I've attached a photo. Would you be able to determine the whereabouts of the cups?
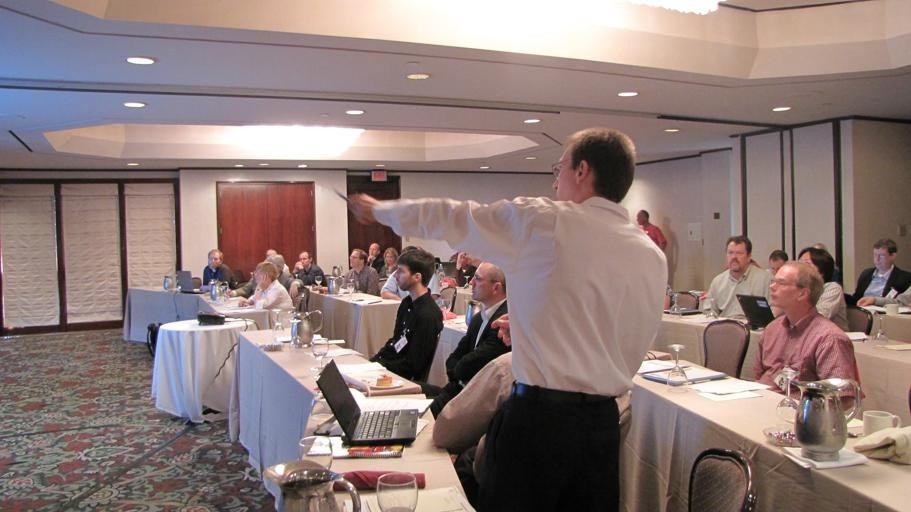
[886,303,902,317]
[863,410,902,438]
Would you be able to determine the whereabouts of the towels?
[782,446,866,468]
[333,473,423,490]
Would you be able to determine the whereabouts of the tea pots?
[465,299,483,327]
[328,276,344,295]
[163,275,174,291]
[291,310,324,349]
[209,279,216,300]
[277,467,364,512]
[332,265,344,278]
[791,377,861,462]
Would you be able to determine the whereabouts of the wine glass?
[216,281,230,305]
[706,297,719,322]
[669,292,682,319]
[668,343,690,395]
[272,308,286,346]
[311,399,333,428]
[873,317,890,348]
[348,283,355,301]
[316,276,322,292]
[376,473,419,512]
[778,367,803,424]
[311,338,328,372]
[463,276,472,291]
[298,435,334,473]
[442,300,452,323]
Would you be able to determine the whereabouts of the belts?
[509,384,610,406]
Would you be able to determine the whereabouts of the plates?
[763,426,802,448]
[369,380,406,388]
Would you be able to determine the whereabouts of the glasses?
[770,280,804,291]
[350,256,364,260]
[552,159,571,179]
[473,274,499,283]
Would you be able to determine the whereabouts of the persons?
[856,284,911,312]
[415,261,518,410]
[202,248,235,291]
[631,207,665,270]
[256,248,287,274]
[289,249,325,288]
[227,256,303,297]
[764,250,792,282]
[851,240,908,306]
[380,244,442,301]
[345,125,664,506]
[365,242,382,273]
[342,248,377,293]
[750,262,862,412]
[702,236,777,326]
[453,247,477,289]
[368,249,442,378]
[797,250,847,331]
[377,247,400,281]
[432,343,632,460]
[239,263,293,334]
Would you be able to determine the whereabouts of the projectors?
[197,311,226,326]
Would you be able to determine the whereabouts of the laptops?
[735,294,775,331]
[316,358,419,446]
[178,272,207,294]
[663,308,703,316]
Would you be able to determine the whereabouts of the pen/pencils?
[332,188,348,201]
[682,378,710,385]
[368,300,382,304]
[352,299,364,301]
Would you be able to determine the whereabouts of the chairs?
[670,291,699,310]
[847,304,873,335]
[192,277,202,288]
[703,319,750,379]
[440,287,457,312]
[377,278,387,290]
[298,286,310,309]
[292,292,307,312]
[688,447,758,511]
[236,281,249,288]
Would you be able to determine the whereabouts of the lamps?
[626,0,728,16]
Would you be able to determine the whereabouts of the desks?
[229,329,422,481]
[698,298,711,311]
[302,394,476,512]
[302,285,402,360]
[620,359,911,511]
[151,318,257,424]
[873,313,911,344]
[123,285,206,344]
[651,309,911,428]
[427,315,469,389]
[439,286,473,315]
[198,294,270,330]
